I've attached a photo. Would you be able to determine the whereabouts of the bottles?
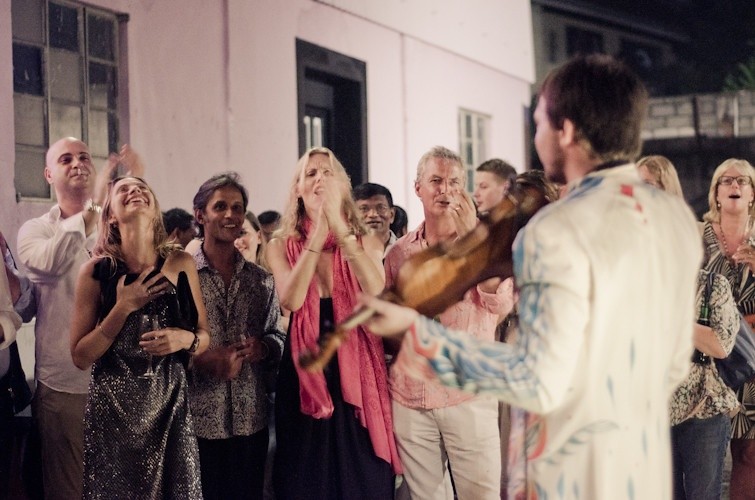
[693,303,711,364]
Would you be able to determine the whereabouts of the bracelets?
[99,325,118,340]
[301,247,324,255]
[335,229,356,242]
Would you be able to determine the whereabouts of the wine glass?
[138,315,162,380]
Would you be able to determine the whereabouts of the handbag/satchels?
[176,271,199,368]
[706,271,755,388]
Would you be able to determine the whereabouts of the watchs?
[185,331,199,352]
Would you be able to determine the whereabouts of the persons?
[357,51,704,500]
[0,134,755,500]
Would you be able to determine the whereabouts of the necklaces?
[717,216,748,265]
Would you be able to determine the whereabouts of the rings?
[147,290,152,295]
[454,207,461,211]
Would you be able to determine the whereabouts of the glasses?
[717,175,754,188]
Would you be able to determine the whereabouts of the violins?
[300,194,548,373]
